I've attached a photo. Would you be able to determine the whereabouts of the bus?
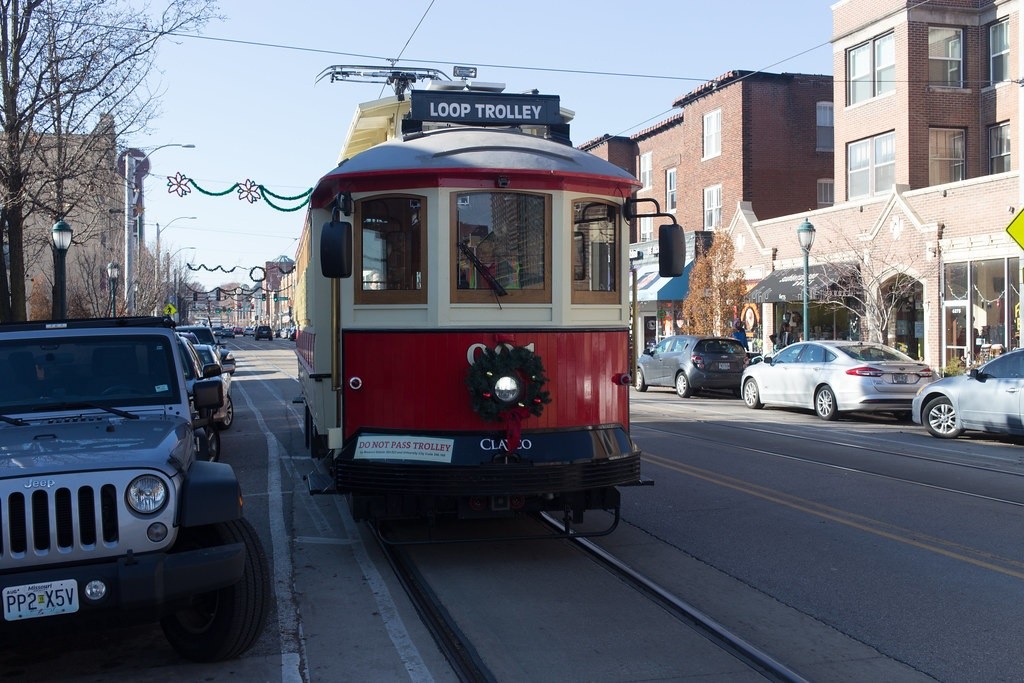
[290,59,685,544]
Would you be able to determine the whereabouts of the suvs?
[0,315,271,663]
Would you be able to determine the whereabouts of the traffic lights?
[215,308,220,315]
[237,303,241,309]
[274,293,278,301]
[251,304,254,309]
[227,307,230,313]
[262,293,266,301]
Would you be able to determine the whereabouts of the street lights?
[173,264,197,324]
[125,143,197,317]
[50,218,72,323]
[796,218,817,341]
[155,216,198,318]
[107,259,119,319]
[166,247,196,314]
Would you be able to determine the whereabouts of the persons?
[732,318,749,352]
[461,242,516,290]
[775,322,793,349]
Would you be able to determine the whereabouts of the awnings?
[628,257,694,302]
[743,262,862,303]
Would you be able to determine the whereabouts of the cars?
[274,326,296,341]
[912,348,1024,439]
[152,322,274,463]
[635,335,752,400]
[741,340,933,421]
